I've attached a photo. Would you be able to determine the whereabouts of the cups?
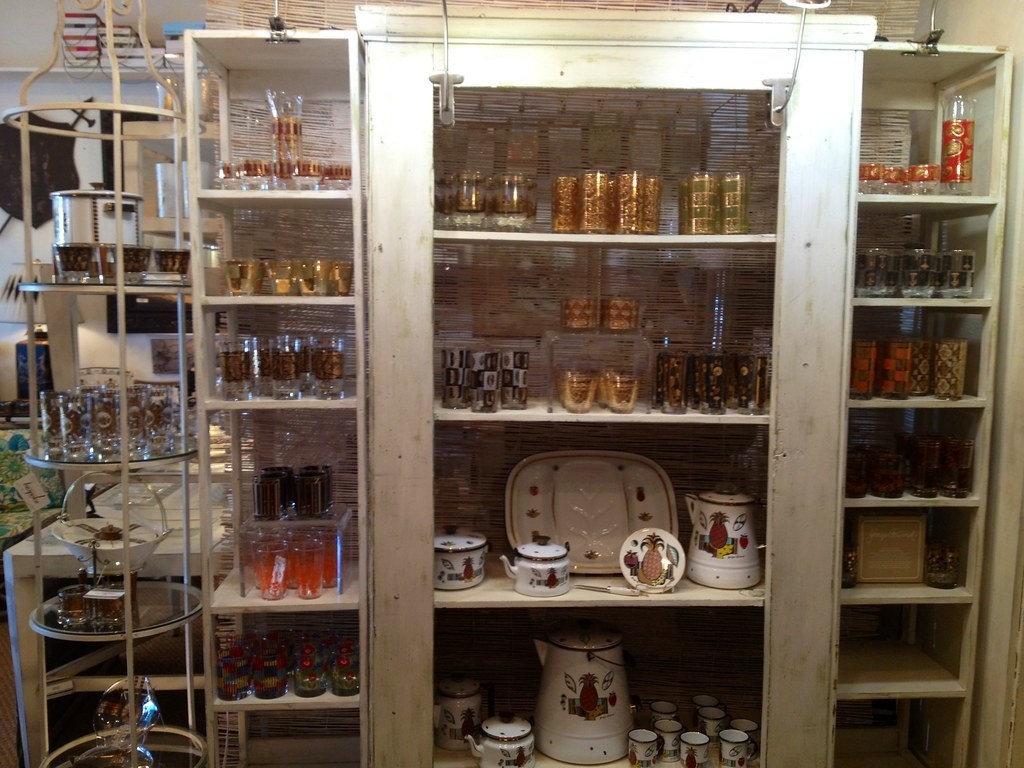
[842,548,858,587]
[214,330,346,400]
[216,626,360,701]
[219,88,351,190]
[852,243,980,297]
[248,465,333,600]
[625,694,762,768]
[56,569,139,631]
[40,379,177,458]
[218,250,355,297]
[923,539,962,590]
[434,164,750,235]
[856,93,979,195]
[52,240,190,286]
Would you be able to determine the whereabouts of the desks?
[3,443,257,768]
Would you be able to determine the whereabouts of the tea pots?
[464,710,536,768]
[683,479,767,590]
[498,536,571,597]
[433,672,493,751]
[529,617,640,765]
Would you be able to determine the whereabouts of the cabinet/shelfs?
[366,34,1013,768]
[0,0,366,768]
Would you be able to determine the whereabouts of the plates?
[620,528,686,594]
[505,450,679,576]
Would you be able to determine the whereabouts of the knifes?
[575,584,641,597]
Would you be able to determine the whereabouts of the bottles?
[848,337,971,400]
[548,294,653,412]
[438,343,769,415]
[846,426,978,501]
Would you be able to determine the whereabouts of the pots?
[434,523,492,590]
[47,181,145,249]
